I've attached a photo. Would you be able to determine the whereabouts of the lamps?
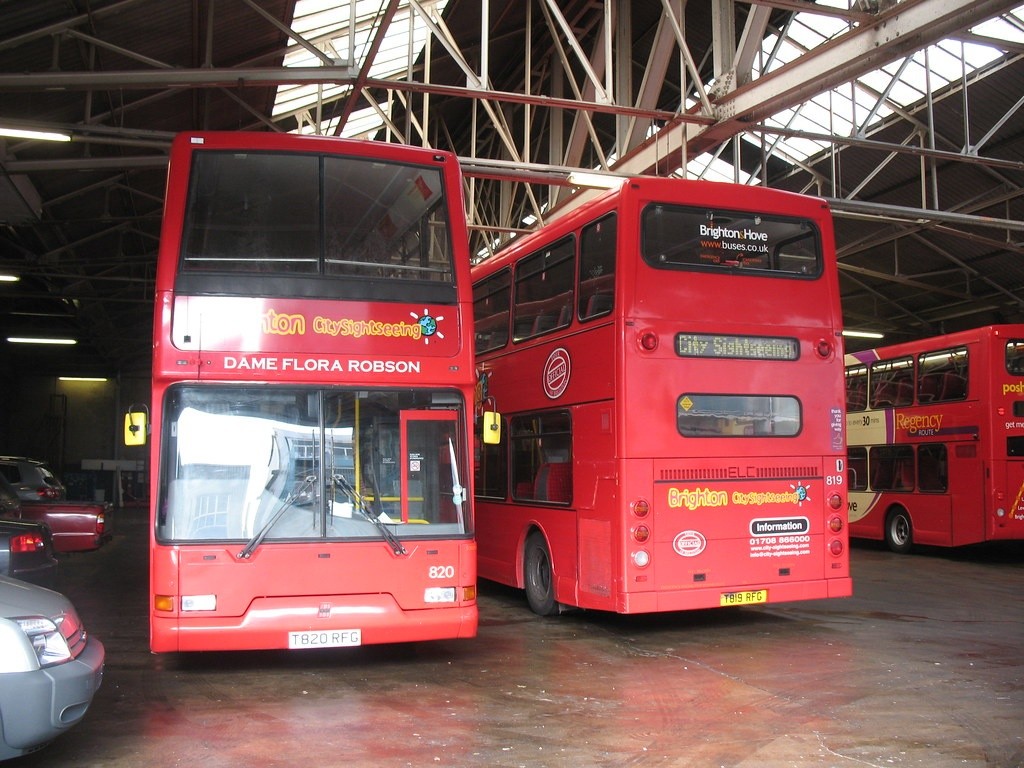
[567,172,625,191]
[0,122,73,142]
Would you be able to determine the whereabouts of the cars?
[0,454,116,762]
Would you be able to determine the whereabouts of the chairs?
[847,455,945,489]
[516,459,573,502]
[475,274,615,352]
[847,371,966,410]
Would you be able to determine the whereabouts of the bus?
[468,177,854,618]
[844,323,1024,556]
[122,130,483,652]
